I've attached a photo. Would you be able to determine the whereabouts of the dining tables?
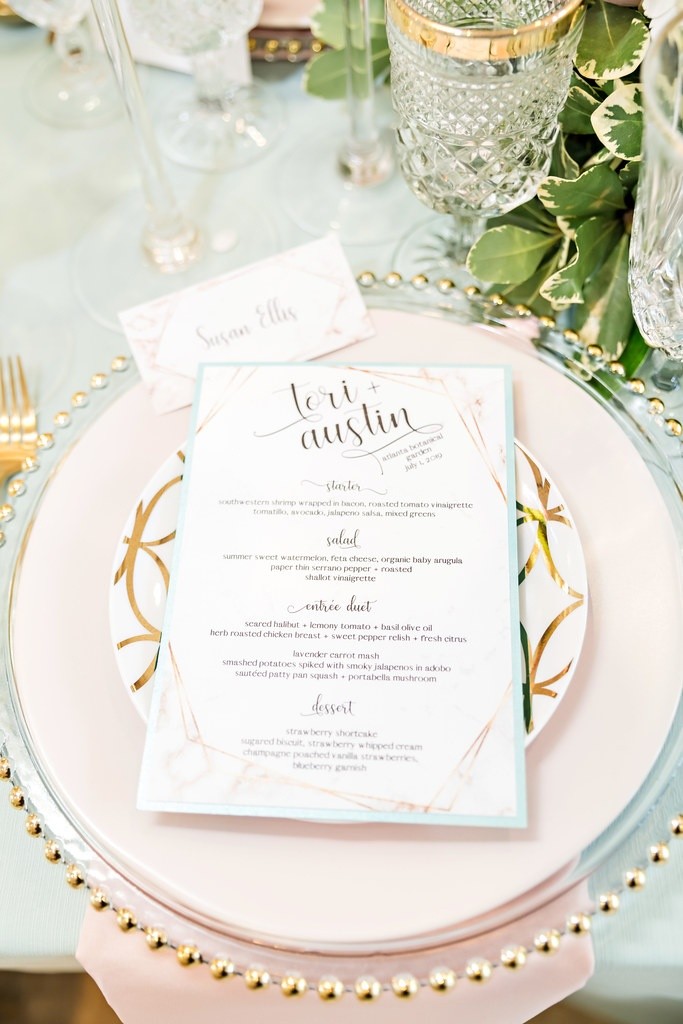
[0,1,683,1024]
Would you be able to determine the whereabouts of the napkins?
[4,1,263,86]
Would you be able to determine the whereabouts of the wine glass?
[4,1,131,128]
[628,0,683,406]
[385,0,589,292]
[123,0,286,173]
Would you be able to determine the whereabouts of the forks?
[0,354,38,495]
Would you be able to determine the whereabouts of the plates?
[7,309,682,960]
[108,436,592,754]
[2,269,682,1003]
